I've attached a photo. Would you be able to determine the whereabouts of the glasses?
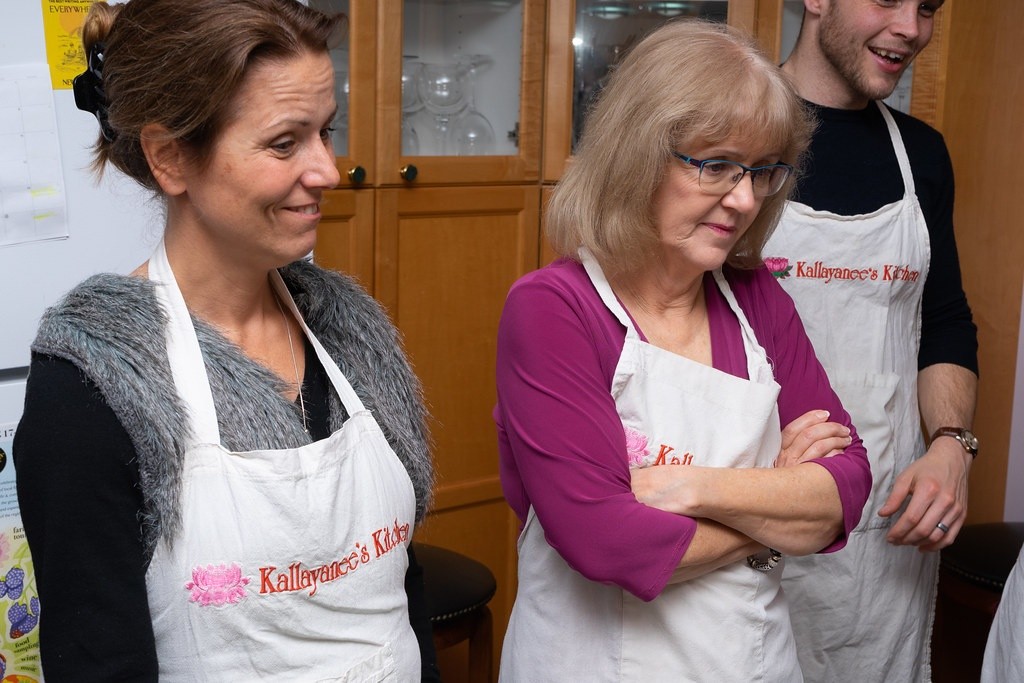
[669,148,795,198]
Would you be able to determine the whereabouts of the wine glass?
[329,52,497,156]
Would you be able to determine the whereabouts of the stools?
[399,543,499,683]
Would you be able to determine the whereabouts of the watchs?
[927,425,978,461]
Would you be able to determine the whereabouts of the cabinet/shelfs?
[294,0,732,683]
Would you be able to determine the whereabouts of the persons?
[11,0,441,683]
[493,13,871,683]
[758,1,977,683]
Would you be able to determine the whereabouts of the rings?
[936,522,949,532]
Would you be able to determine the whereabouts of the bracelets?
[746,548,781,573]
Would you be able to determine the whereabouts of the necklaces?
[269,289,311,435]
